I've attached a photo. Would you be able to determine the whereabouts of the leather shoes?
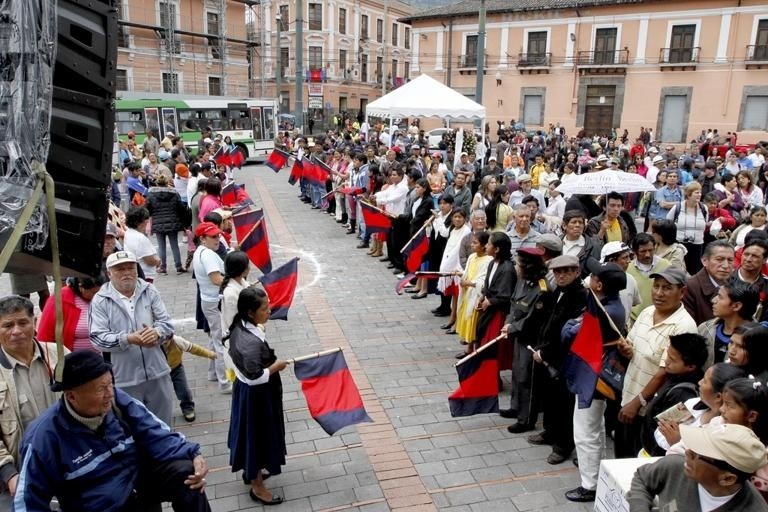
[431,306,469,359]
[249,487,283,505]
[242,468,271,484]
[565,486,596,501]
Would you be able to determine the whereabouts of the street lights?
[276,10,281,97]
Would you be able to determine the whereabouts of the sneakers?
[184,410,195,422]
[298,194,428,299]
[547,446,572,464]
[527,431,551,444]
[208,375,218,381]
[499,408,520,420]
[220,384,233,394]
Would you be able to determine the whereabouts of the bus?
[112,96,281,165]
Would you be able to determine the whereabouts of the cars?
[277,113,295,130]
[424,128,455,149]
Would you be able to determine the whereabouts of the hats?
[106,250,139,269]
[611,158,620,165]
[548,255,580,270]
[597,154,609,162]
[430,152,441,158]
[159,150,172,161]
[679,423,768,473]
[204,125,220,145]
[516,174,532,186]
[652,155,666,165]
[533,233,563,252]
[488,157,496,162]
[51,349,112,392]
[587,257,627,290]
[647,146,658,154]
[128,130,175,138]
[599,241,629,263]
[105,224,117,237]
[516,247,544,259]
[648,265,689,286]
[460,152,468,158]
[194,222,222,237]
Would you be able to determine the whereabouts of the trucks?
[695,134,756,157]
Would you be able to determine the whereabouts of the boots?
[156,264,168,276]
[175,264,189,275]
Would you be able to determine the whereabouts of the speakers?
[0,0,119,94]
[0,181,110,280]
[0,81,114,185]
